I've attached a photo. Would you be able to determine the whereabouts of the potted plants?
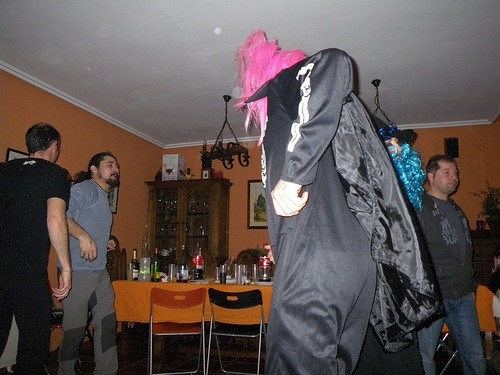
[469,180,500,229]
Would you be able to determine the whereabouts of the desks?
[111,277,274,375]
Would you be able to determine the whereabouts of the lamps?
[200,94,252,170]
[367,79,419,145]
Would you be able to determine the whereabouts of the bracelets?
[62,268,73,273]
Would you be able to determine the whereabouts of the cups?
[233,263,248,285]
[250,263,259,282]
[127,263,134,281]
[169,264,177,282]
[219,264,227,284]
[157,198,208,235]
[176,263,189,282]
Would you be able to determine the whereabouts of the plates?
[190,277,275,284]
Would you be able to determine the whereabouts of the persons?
[56,152,119,375]
[418,154,485,375]
[0,122,71,375]
[105,235,120,282]
[372,115,442,375]
[231,30,439,375]
[442,249,500,375]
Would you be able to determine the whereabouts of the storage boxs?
[200,170,224,179]
[161,154,183,180]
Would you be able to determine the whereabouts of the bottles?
[259,243,272,282]
[151,248,161,283]
[139,243,153,282]
[130,248,140,281]
[193,247,203,281]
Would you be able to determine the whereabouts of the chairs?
[145,286,206,375]
[206,287,267,375]
[43,304,95,375]
[436,284,496,375]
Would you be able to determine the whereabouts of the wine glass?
[213,266,220,282]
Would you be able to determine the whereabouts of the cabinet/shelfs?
[470,229,500,294]
[143,179,235,275]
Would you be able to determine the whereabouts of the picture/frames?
[6,148,29,161]
[247,179,271,229]
[108,185,120,214]
